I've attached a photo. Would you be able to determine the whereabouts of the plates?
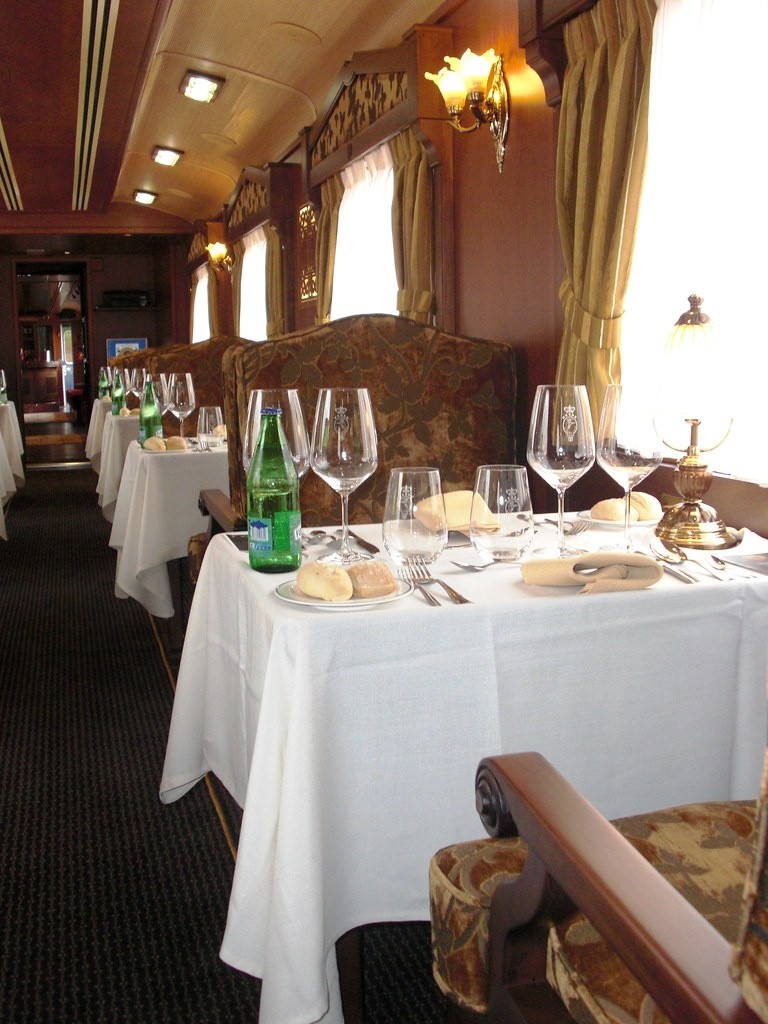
[274,578,413,612]
[146,450,186,452]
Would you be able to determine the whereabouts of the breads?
[143,436,188,451]
[213,424,227,439]
[119,408,140,417]
[296,560,397,600]
[102,396,110,400]
[590,492,663,522]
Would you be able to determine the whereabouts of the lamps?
[132,189,160,207]
[650,294,741,550]
[204,242,231,272]
[150,144,184,168]
[423,48,509,175]
[177,69,226,104]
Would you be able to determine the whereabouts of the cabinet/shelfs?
[19,315,65,412]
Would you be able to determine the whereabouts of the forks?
[543,516,594,536]
[397,568,441,606]
[201,442,208,451]
[407,556,469,604]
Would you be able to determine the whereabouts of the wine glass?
[241,389,310,564]
[166,373,196,449]
[526,384,595,559]
[309,388,378,566]
[595,384,663,554]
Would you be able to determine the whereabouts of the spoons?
[451,559,498,573]
[312,530,337,542]
[650,538,725,582]
[707,553,751,579]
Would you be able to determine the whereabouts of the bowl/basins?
[575,509,665,532]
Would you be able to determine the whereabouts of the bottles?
[246,408,302,573]
[98,370,109,399]
[139,382,163,448]
[111,374,126,415]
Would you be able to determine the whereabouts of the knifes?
[337,529,379,554]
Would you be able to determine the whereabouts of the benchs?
[434,736,768,1024]
[108,311,530,588]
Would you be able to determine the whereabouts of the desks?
[93,409,141,525]
[103,433,229,619]
[154,506,768,1024]
[0,401,26,540]
[84,396,112,475]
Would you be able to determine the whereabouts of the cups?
[197,406,223,446]
[470,464,534,563]
[383,466,448,566]
[100,366,170,416]
